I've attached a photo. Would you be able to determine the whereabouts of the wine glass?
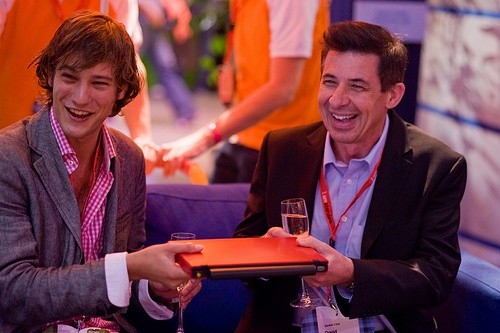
[171,232,196,333]
[281,198,323,307]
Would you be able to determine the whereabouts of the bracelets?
[207,121,222,143]
[342,274,354,293]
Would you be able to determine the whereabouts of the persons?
[0,0,201,184]
[233,20,468,333]
[0,8,202,333]
[158,0,331,184]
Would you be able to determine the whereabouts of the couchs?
[125,183,500,333]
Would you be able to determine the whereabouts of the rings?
[176,283,184,292]
